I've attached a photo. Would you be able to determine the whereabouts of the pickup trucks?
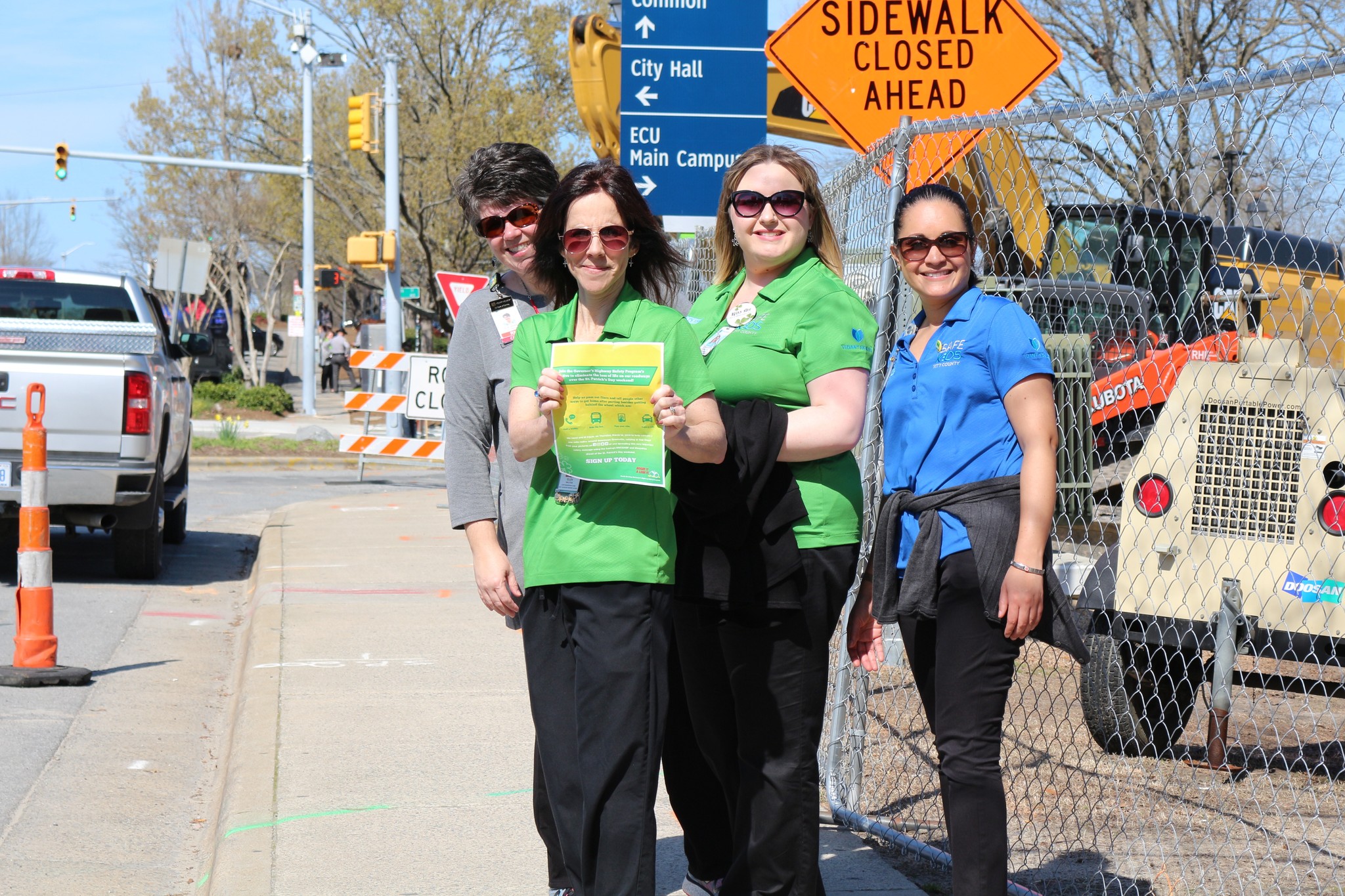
[0,265,212,579]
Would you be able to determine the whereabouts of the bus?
[148,258,243,363]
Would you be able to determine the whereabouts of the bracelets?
[1010,560,1045,575]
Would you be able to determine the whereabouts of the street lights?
[1212,150,1246,228]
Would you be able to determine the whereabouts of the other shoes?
[548,887,574,896]
[667,871,722,896]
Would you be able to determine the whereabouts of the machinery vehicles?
[572,15,1345,506]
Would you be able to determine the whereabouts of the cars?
[244,318,283,356]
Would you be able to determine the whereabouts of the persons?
[319,303,333,332]
[508,159,727,896]
[846,184,1059,896]
[329,328,361,392]
[662,144,877,896]
[445,142,690,896]
[320,330,336,393]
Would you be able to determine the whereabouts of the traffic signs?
[619,0,770,235]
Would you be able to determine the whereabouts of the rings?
[670,406,675,415]
[534,390,544,400]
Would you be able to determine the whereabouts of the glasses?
[476,203,546,239]
[560,224,639,255]
[897,231,974,262]
[725,190,813,218]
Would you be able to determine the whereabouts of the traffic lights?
[347,93,371,153]
[320,269,340,288]
[54,143,69,180]
[381,234,395,261]
[71,206,78,220]
